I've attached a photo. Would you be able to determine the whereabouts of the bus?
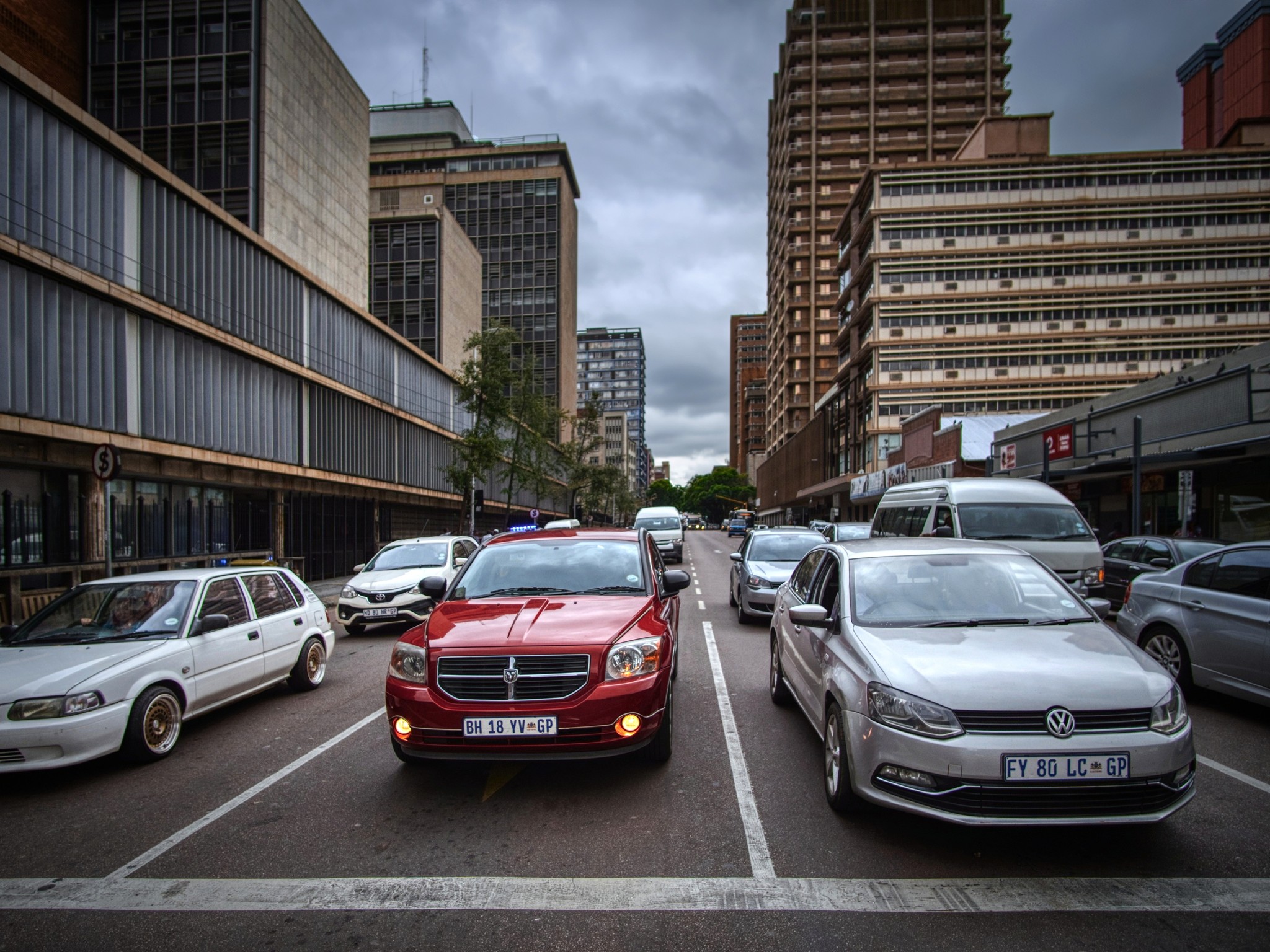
[733,510,756,532]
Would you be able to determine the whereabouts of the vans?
[870,477,1111,619]
[544,519,582,529]
[633,506,687,563]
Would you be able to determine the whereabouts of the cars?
[383,527,691,768]
[1100,535,1226,609]
[754,520,873,542]
[768,537,1199,826]
[0,566,334,776]
[1113,541,1270,707]
[688,521,720,530]
[336,535,480,635]
[721,519,729,531]
[729,528,830,624]
[1218,494,1270,540]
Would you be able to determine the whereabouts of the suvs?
[728,519,748,537]
